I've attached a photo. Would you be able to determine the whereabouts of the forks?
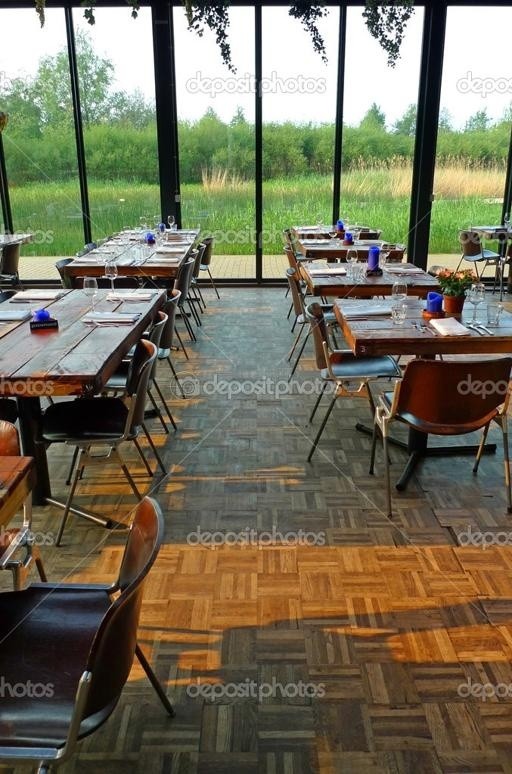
[420,320,438,336]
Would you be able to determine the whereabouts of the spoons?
[410,319,427,333]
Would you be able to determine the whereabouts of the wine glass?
[328,225,339,248]
[153,215,161,230]
[139,216,148,231]
[345,248,358,278]
[391,281,408,307]
[316,213,325,231]
[83,277,99,319]
[97,225,131,264]
[466,282,486,325]
[104,262,119,295]
[504,212,512,225]
[158,222,166,242]
[167,215,175,231]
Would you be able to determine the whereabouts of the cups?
[389,304,408,325]
[134,227,141,243]
[351,265,364,281]
[135,243,151,261]
[486,304,503,325]
